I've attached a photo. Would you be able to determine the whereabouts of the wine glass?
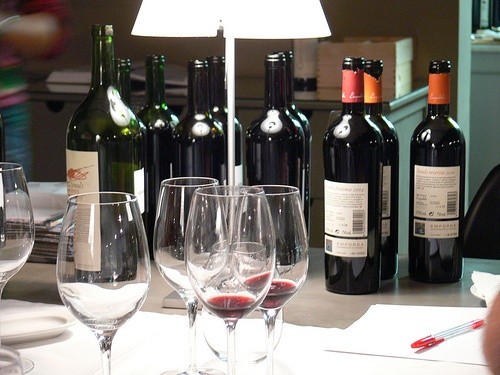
[183,186,276,375]
[232,184,310,374]
[152,177,224,375]
[0,162,35,375]
[56,192,152,375]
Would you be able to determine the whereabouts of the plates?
[0,311,71,345]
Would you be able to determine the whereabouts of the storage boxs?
[316,36,414,101]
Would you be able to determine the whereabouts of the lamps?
[130,0,332,239]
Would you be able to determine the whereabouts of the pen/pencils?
[411,318,485,349]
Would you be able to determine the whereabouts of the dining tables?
[0,246,500,375]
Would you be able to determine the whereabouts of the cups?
[201,241,284,367]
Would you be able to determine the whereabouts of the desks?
[28,64,429,246]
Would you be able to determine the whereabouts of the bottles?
[321,56,383,296]
[114,53,311,277]
[64,24,138,284]
[362,58,400,281]
[409,59,466,285]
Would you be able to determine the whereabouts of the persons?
[0,0,69,185]
